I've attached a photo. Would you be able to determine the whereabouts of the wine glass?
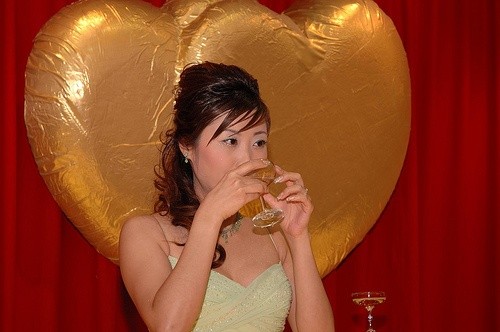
[352,292,387,332]
[242,159,285,229]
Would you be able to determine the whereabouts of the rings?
[302,186,309,196]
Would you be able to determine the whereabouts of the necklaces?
[217,212,244,240]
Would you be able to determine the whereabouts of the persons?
[116,58,339,331]
